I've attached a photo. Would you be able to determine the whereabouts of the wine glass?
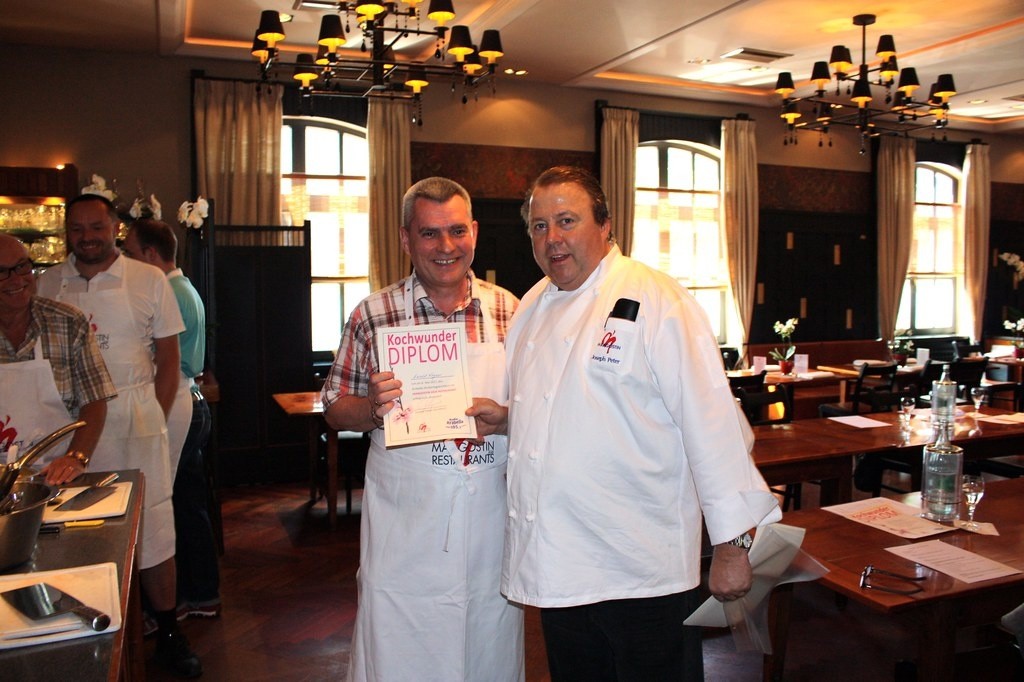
[901,397,915,422]
[960,474,985,530]
[970,387,985,418]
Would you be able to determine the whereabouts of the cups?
[753,356,767,372]
[916,348,930,367]
[0,204,67,279]
[794,354,809,375]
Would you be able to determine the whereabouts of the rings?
[374,399,382,406]
[69,466,74,470]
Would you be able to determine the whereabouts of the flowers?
[878,336,914,350]
[1003,319,1024,346]
[767,316,800,360]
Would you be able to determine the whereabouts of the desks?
[267,353,1022,681]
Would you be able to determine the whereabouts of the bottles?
[920,365,964,522]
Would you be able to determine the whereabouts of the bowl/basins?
[0,464,60,570]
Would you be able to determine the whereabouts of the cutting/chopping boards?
[41,481,134,525]
[0,561,122,648]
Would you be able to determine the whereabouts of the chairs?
[903,355,962,405]
[817,362,898,416]
[949,356,989,403]
[730,383,789,425]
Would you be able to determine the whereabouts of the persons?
[125,219,219,633]
[466,166,782,682]
[0,233,119,484]
[319,177,522,682]
[37,194,201,682]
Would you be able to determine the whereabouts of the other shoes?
[143,601,187,635]
[187,594,220,617]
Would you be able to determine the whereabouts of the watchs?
[725,533,754,549]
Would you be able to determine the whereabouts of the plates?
[853,359,887,366]
[751,365,782,371]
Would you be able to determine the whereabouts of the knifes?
[42,519,105,527]
[1,582,111,631]
[53,473,119,511]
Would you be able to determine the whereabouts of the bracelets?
[65,450,90,468]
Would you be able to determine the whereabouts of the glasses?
[859,564,928,594]
[0,257,34,282]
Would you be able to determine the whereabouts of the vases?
[893,352,909,364]
[1013,347,1024,358]
[777,359,793,375]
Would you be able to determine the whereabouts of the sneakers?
[154,633,205,677]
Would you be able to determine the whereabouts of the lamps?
[249,0,504,127]
[774,13,958,157]
